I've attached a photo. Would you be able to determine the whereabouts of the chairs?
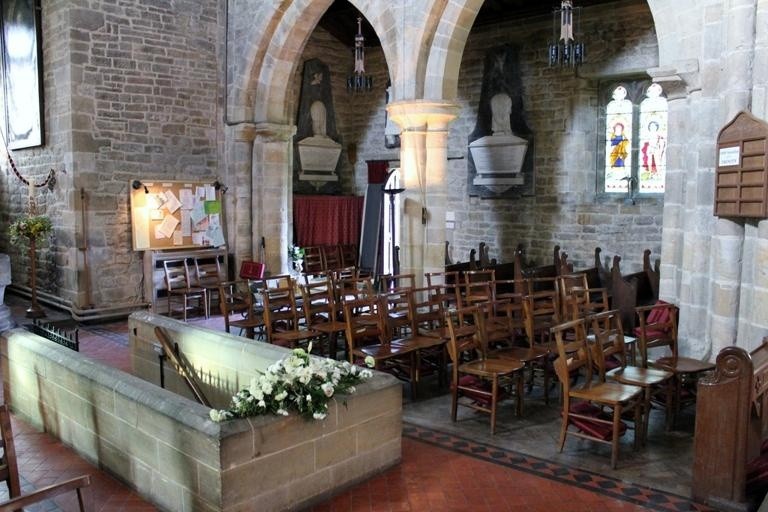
[0,406,25,512]
[0,475,92,512]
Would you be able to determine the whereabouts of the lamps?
[549,0,588,67]
[346,17,373,93]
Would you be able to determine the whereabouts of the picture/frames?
[0,0,45,151]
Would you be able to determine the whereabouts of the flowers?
[6,215,53,246]
